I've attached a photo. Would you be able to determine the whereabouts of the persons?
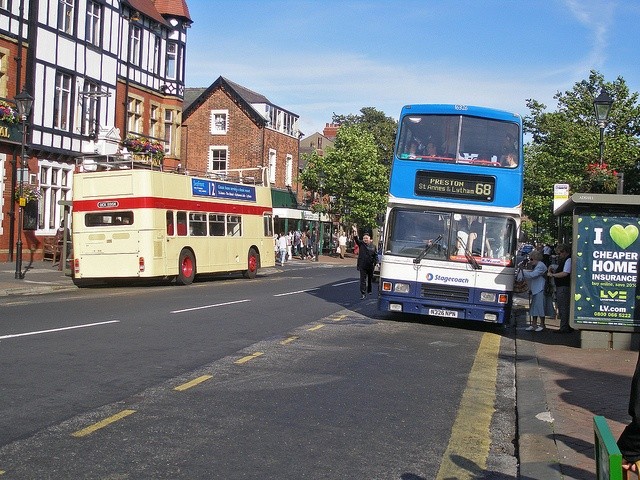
[518,251,549,333]
[547,243,575,335]
[339,234,347,259]
[278,232,288,267]
[288,227,338,268]
[454,220,468,256]
[351,226,379,301]
[467,221,493,259]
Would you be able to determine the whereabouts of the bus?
[378,104,523,323]
[72,152,275,287]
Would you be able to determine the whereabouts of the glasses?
[530,258,534,261]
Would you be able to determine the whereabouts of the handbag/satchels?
[513,264,528,294]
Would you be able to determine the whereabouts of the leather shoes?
[568,328,574,332]
[525,325,538,331]
[535,325,546,331]
[553,328,567,334]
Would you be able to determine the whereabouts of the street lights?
[594,88,614,170]
[13,85,34,279]
